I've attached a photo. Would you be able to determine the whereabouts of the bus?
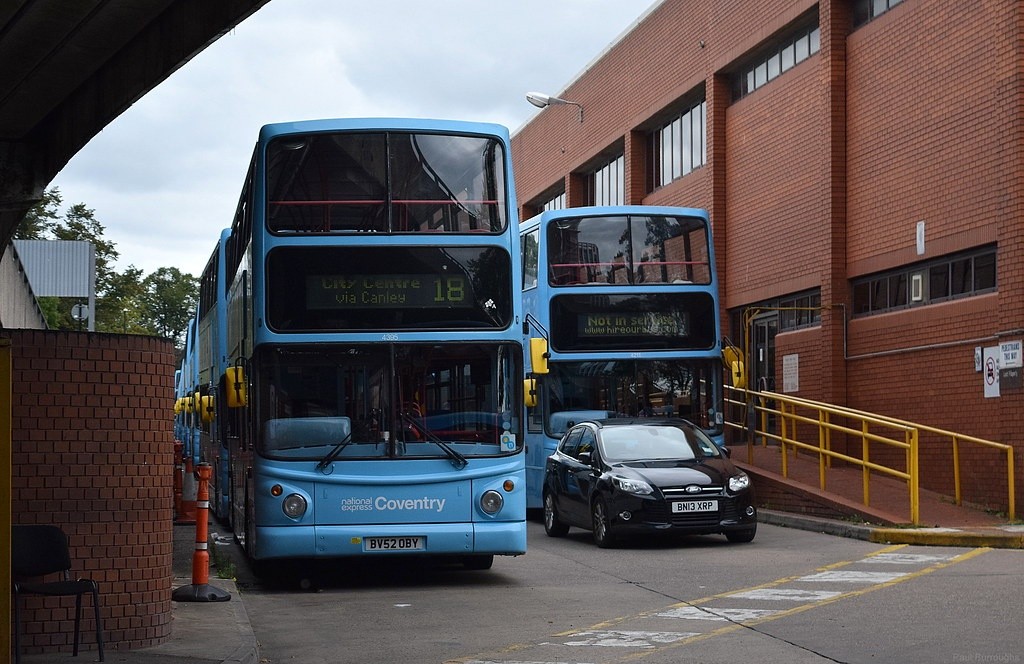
[430,207,745,510]
[175,118,549,570]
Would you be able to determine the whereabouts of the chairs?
[10,523,105,664]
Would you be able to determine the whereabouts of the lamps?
[526,92,583,123]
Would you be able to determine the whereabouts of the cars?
[542,418,758,547]
